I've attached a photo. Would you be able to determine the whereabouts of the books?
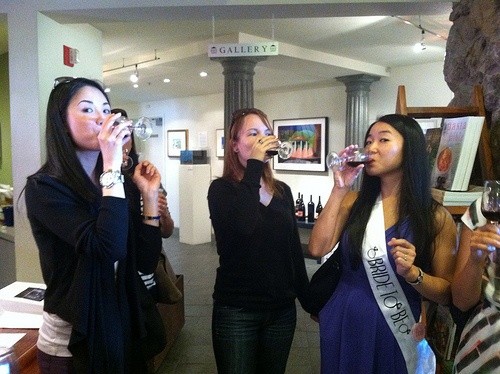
[430,115,485,191]
[431,184,492,207]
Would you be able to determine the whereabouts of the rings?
[403,253,408,260]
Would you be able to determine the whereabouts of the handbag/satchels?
[308,250,344,317]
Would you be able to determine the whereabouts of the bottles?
[316,195,322,220]
[296,193,300,218]
[298,194,305,221]
[308,195,314,222]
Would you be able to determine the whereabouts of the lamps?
[103,83,110,93]
[130,64,139,83]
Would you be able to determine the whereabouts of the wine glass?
[326,147,370,170]
[112,116,152,142]
[120,148,133,171]
[266,138,293,159]
[481,180,500,252]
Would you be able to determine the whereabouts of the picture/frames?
[216,128,225,157]
[166,130,188,157]
[272,116,328,172]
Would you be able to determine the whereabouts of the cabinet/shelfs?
[294,216,325,264]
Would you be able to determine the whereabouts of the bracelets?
[406,267,425,287]
[141,214,161,221]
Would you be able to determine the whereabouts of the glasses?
[229,108,267,139]
[53,76,104,90]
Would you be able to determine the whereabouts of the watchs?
[99,168,125,189]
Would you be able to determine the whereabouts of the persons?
[450,189,500,374]
[110,108,174,239]
[15,74,162,374]
[205,106,325,374]
[308,111,457,374]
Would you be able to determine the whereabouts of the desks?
[0,327,42,374]
